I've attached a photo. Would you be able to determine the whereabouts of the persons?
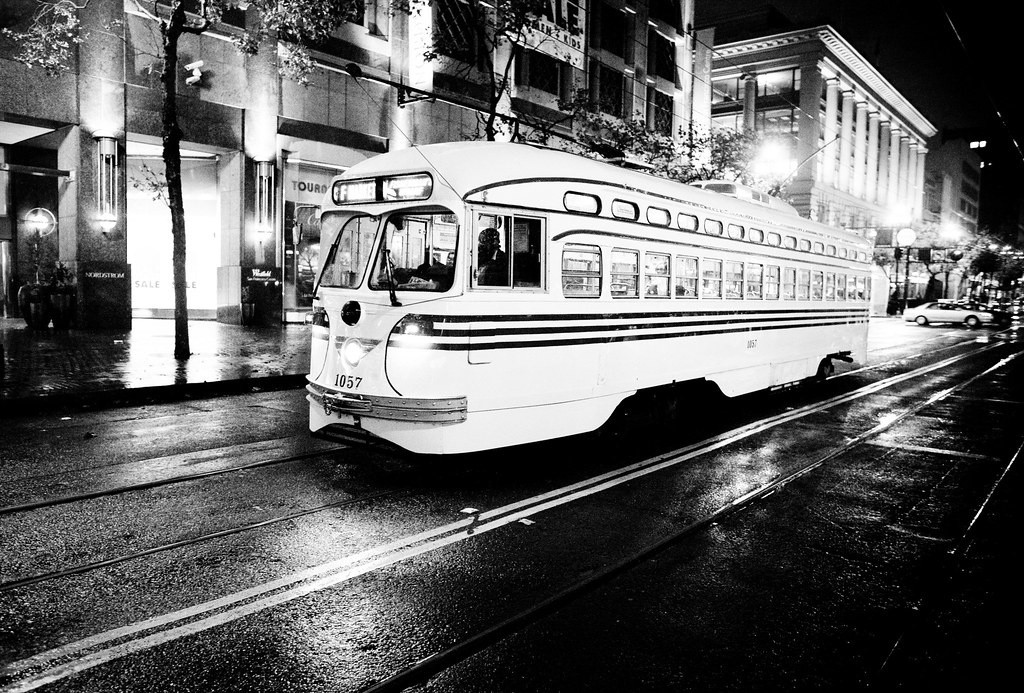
[446,252,455,267]
[477,228,508,287]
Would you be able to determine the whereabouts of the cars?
[962,295,1024,328]
[902,301,994,329]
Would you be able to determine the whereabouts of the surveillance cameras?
[184,60,204,71]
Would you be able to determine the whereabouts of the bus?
[305,143,874,468]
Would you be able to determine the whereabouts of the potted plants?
[18,229,74,334]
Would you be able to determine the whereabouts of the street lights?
[896,227,917,314]
